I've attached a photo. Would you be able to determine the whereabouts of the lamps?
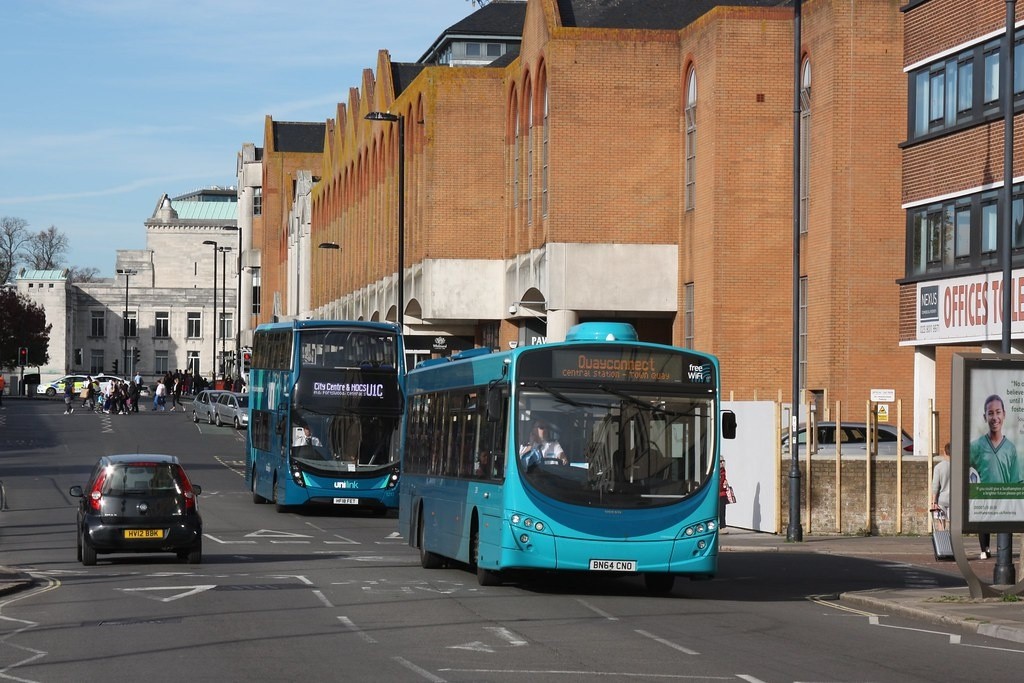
[318,243,342,250]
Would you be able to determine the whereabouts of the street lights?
[212,247,232,380]
[364,111,404,329]
[116,269,137,373]
[202,240,217,390]
[223,225,242,392]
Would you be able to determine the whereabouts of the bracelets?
[934,502,937,504]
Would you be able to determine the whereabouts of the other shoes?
[71,409,75,414]
[64,411,69,415]
[985,546,990,553]
[719,528,729,534]
[152,408,157,411]
[81,402,140,415]
[169,408,176,412]
[981,552,987,560]
[182,404,186,412]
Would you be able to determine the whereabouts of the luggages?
[929,508,955,562]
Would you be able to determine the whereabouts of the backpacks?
[520,448,544,473]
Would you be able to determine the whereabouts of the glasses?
[537,424,551,430]
[719,460,725,463]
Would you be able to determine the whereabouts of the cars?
[191,381,249,430]
[140,385,152,397]
[69,454,202,566]
[782,422,913,455]
[37,373,130,397]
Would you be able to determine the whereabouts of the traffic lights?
[18,347,28,366]
[230,350,236,368]
[112,359,118,374]
[75,349,81,365]
[244,353,251,373]
[135,347,141,363]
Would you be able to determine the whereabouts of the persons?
[163,368,209,395]
[134,372,142,392]
[169,377,186,411]
[477,449,490,474]
[970,394,1019,484]
[234,375,246,392]
[63,381,74,414]
[0,373,6,406]
[295,425,322,446]
[719,455,729,534]
[223,375,234,390]
[519,420,569,466]
[932,443,951,531]
[151,380,166,411]
[150,468,173,487]
[81,376,140,414]
[979,533,990,560]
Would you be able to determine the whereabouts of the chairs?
[632,441,664,480]
[370,360,379,367]
[359,360,373,369]
[379,360,393,368]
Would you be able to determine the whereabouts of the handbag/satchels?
[157,394,166,405]
[79,388,89,398]
[723,480,736,503]
[124,389,129,400]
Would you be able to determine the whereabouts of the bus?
[398,322,738,587]
[245,320,406,514]
